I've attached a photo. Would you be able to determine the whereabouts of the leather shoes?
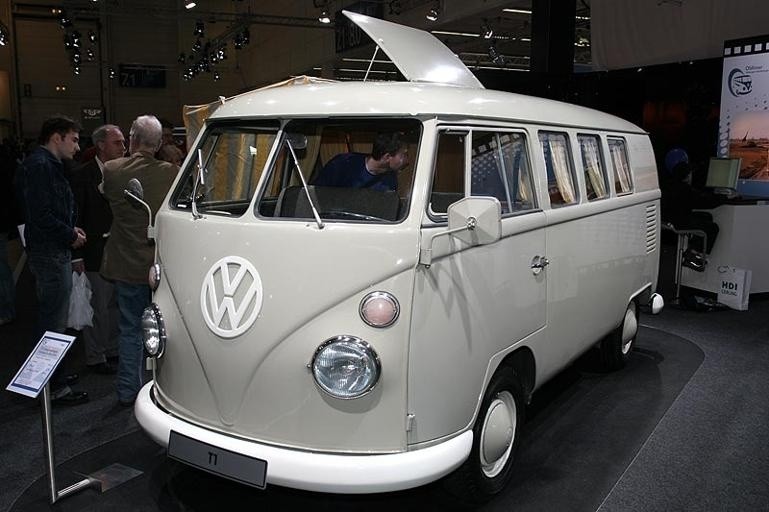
[49,370,90,407]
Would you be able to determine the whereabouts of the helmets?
[662,148,688,178]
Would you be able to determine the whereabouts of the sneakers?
[681,249,709,273]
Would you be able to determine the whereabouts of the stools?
[660,221,710,307]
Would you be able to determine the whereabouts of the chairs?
[270,182,486,224]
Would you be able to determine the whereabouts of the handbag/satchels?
[66,269,97,333]
[716,264,753,313]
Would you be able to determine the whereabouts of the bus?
[132,10,666,498]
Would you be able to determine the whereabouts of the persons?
[313,131,409,192]
[104,115,182,406]
[1,143,16,326]
[70,125,127,374]
[160,144,184,167]
[154,120,175,160]
[18,115,89,406]
[659,163,737,271]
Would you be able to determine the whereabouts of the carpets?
[7,321,745,512]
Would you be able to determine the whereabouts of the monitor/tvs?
[704,157,741,194]
